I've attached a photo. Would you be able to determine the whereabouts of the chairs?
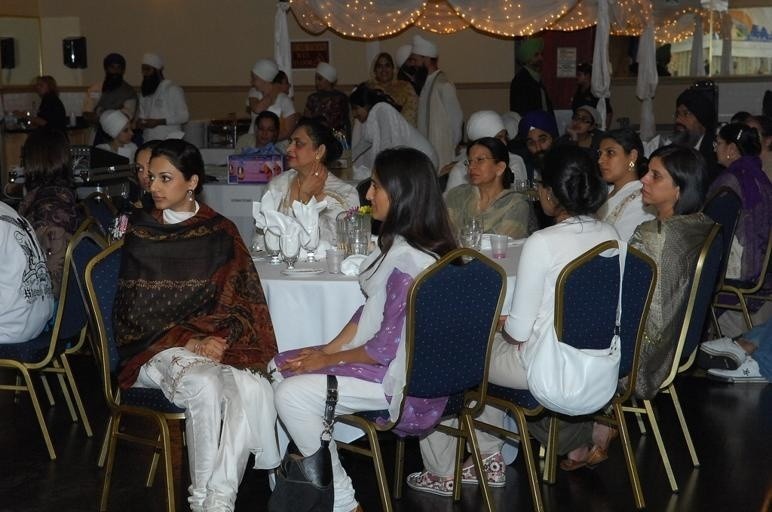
[0,148,771,512]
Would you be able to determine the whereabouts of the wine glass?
[251,226,322,276]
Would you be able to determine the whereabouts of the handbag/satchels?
[267,373,339,512]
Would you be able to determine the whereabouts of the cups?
[488,234,508,259]
[327,248,345,273]
[459,215,482,255]
[516,177,540,198]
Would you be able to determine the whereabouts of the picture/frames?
[289,38,331,71]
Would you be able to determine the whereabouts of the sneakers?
[406,469,455,497]
[557,414,620,472]
[461,451,507,488]
[700,336,748,368]
[706,355,769,384]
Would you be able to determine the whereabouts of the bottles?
[4,102,40,127]
[69,112,77,126]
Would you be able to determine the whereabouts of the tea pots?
[335,209,371,256]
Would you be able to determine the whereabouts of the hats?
[251,58,279,83]
[466,110,506,142]
[502,110,521,141]
[315,61,338,84]
[515,38,544,64]
[574,105,602,129]
[517,113,555,147]
[103,53,127,75]
[411,34,440,58]
[394,44,413,70]
[141,52,165,71]
[99,109,129,138]
[675,88,713,130]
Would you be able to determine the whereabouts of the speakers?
[0,37,15,69]
[62,36,87,68]
